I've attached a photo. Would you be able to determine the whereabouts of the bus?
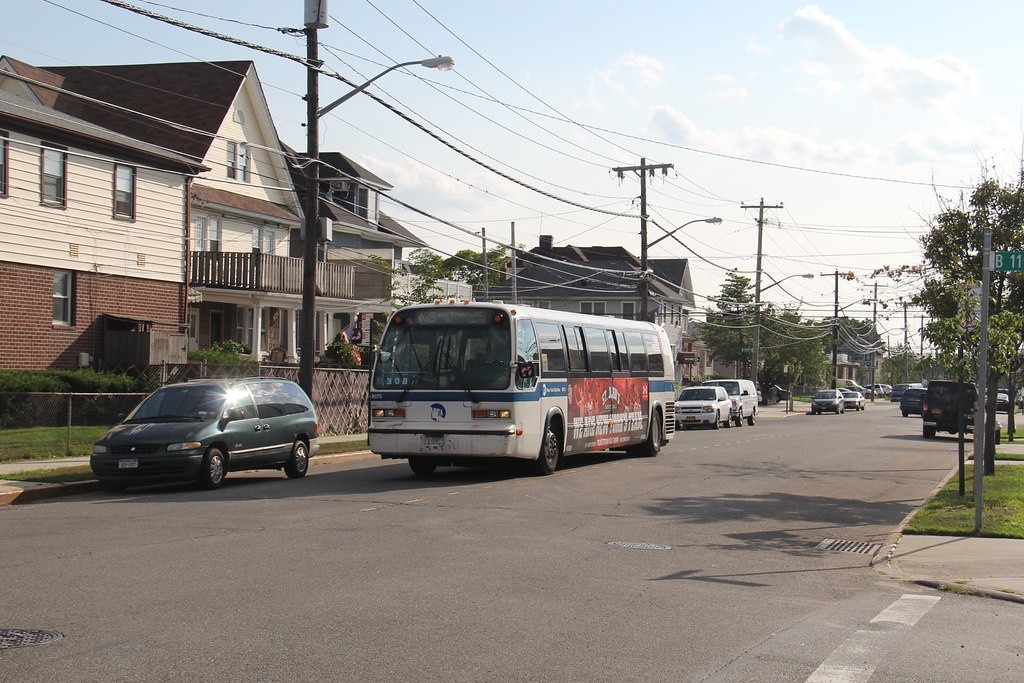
[354,300,676,475]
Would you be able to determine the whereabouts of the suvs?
[675,385,733,429]
[923,379,979,437]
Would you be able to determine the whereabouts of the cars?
[846,387,877,399]
[900,388,928,417]
[842,392,866,411]
[998,389,1009,394]
[866,384,892,395]
[756,382,790,405]
[809,389,845,415]
[984,393,1010,413]
[837,388,852,393]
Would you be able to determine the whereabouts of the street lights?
[638,217,722,319]
[752,272,814,384]
[870,328,904,402]
[297,54,455,397]
[832,298,867,388]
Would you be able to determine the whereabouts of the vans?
[701,380,759,427]
[90,376,320,488]
[891,383,923,402]
